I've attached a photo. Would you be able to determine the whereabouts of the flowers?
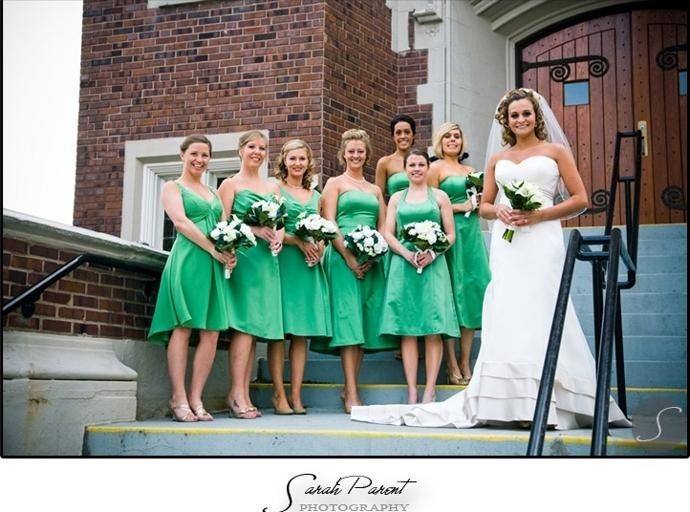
[400,218,450,254]
[344,224,388,261]
[497,179,541,242]
[296,210,338,242]
[247,199,286,231]
[211,220,262,254]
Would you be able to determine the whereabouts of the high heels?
[272,397,295,414]
[168,397,199,422]
[193,403,213,421]
[339,390,366,414]
[287,394,306,414]
[225,395,262,419]
[445,364,470,385]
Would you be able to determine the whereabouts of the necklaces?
[286,180,304,189]
[344,171,366,184]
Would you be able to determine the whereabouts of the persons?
[319,127,388,415]
[160,133,238,422]
[218,130,286,422]
[464,87,633,432]
[428,121,491,387]
[384,148,457,405]
[374,114,430,361]
[268,137,326,417]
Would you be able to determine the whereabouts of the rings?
[525,219,528,224]
